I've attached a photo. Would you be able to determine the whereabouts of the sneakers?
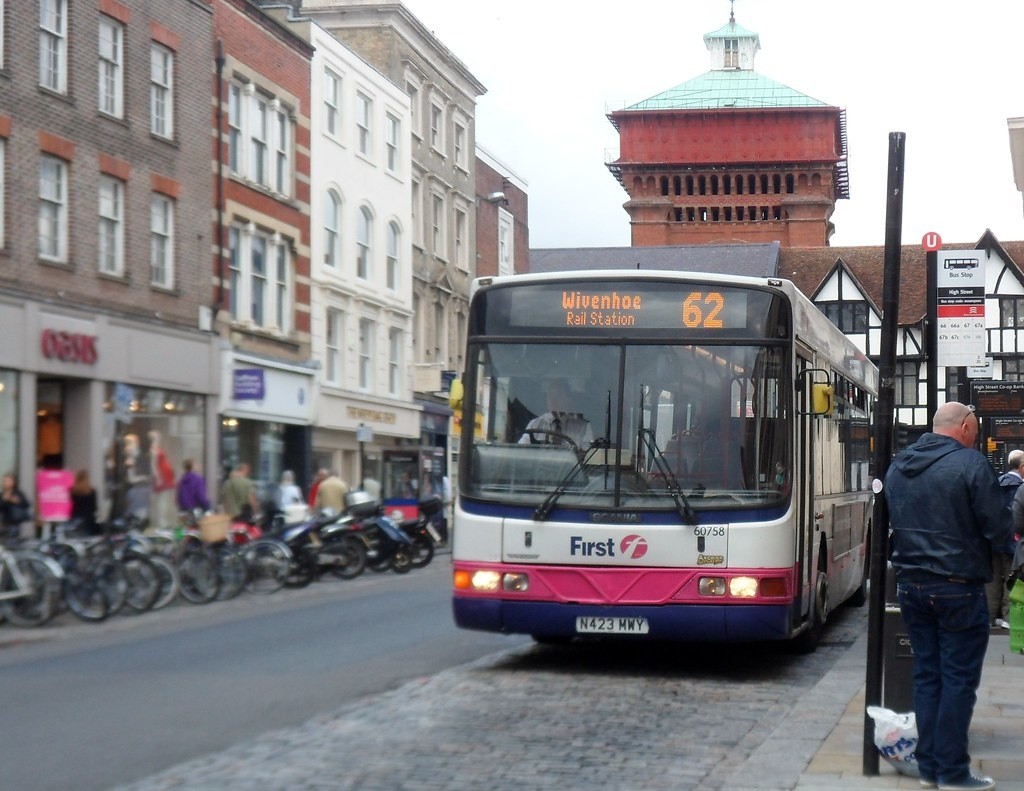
[920,773,996,791]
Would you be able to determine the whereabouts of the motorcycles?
[188,491,444,588]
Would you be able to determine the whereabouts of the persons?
[518,379,595,451]
[0,472,30,524]
[177,459,212,512]
[986,450,1024,630]
[222,464,258,517]
[147,431,175,527]
[68,470,98,532]
[883,402,1013,791]
[124,435,149,520]
[276,468,381,517]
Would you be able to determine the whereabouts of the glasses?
[961,405,976,427]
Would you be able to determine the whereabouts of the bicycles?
[0,488,293,628]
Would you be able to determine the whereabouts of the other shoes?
[1000,621,1011,630]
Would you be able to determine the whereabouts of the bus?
[452,269,879,659]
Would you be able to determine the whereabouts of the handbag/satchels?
[867,706,920,778]
[243,505,254,519]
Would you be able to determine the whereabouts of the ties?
[553,419,562,444]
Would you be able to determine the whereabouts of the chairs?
[648,429,739,491]
